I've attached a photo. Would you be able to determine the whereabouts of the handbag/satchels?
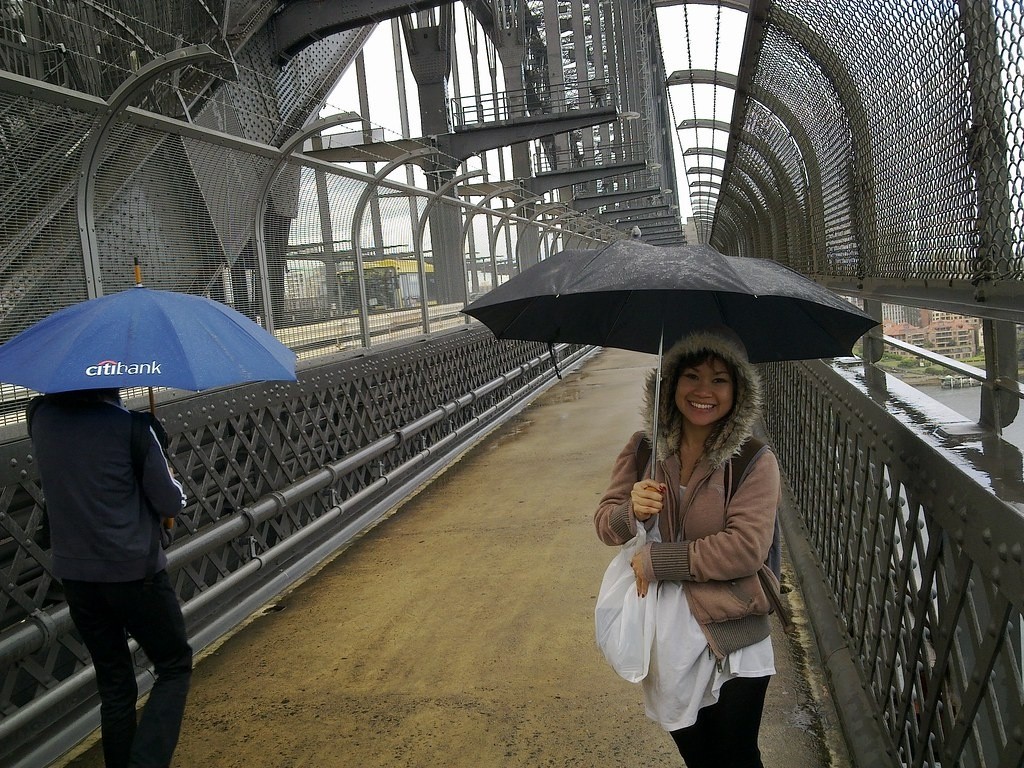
[592,513,661,683]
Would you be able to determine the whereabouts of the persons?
[594,321,782,768]
[25,387,193,768]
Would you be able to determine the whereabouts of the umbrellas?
[0,255,299,416]
[459,239,883,482]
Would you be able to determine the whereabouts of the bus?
[335,260,437,332]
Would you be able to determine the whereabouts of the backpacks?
[635,429,780,615]
[25,395,170,555]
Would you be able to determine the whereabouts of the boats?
[939,375,983,389]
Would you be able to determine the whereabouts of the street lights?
[619,226,641,239]
